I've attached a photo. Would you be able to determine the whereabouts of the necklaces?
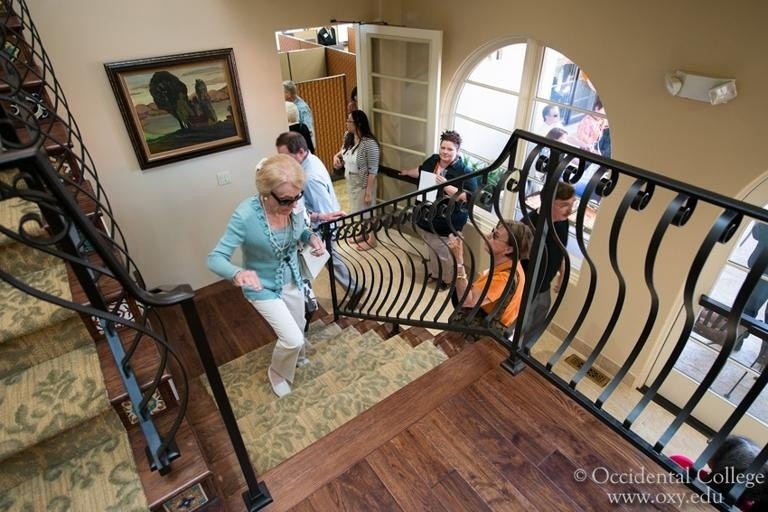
[261,195,293,255]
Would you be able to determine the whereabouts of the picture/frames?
[102,47,258,172]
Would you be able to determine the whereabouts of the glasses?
[271,191,304,205]
[492,228,508,244]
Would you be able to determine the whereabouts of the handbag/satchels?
[333,158,344,176]
[448,307,508,341]
[299,244,330,281]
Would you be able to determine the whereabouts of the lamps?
[666,69,738,104]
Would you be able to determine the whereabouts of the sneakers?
[348,288,366,310]
[428,273,451,292]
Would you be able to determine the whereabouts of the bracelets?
[457,273,467,281]
[308,211,321,222]
[457,264,464,268]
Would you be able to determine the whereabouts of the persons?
[735,219,768,366]
[283,80,316,155]
[333,111,379,251]
[448,218,534,343]
[347,87,358,114]
[303,207,346,338]
[670,436,768,512]
[318,25,336,46]
[285,100,314,154]
[276,132,368,311]
[536,97,613,218]
[498,181,577,351]
[206,154,326,399]
[399,129,478,292]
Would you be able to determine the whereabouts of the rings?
[448,241,454,245]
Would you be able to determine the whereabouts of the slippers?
[349,235,377,251]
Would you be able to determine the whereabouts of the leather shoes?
[296,359,309,368]
[267,366,290,398]
